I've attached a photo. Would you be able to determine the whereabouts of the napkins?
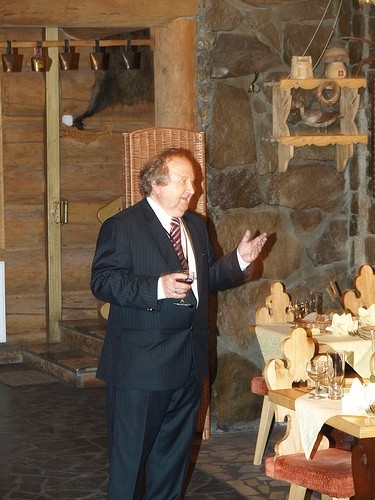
[341,377,375,414]
[358,304,375,327]
[325,312,359,336]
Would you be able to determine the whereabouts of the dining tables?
[268,376,375,441]
[249,306,375,355]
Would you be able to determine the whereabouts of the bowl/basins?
[298,320,332,330]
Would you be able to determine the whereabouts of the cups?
[327,350,345,399]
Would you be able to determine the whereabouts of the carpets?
[0,368,64,389]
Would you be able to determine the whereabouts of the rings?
[175,288,178,294]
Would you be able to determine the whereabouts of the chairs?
[252,262,375,499]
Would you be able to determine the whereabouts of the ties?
[169,217,189,272]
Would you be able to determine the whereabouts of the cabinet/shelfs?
[263,77,368,174]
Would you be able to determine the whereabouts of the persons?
[89,148,268,500]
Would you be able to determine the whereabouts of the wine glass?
[290,301,304,329]
[174,270,194,305]
[305,361,327,399]
